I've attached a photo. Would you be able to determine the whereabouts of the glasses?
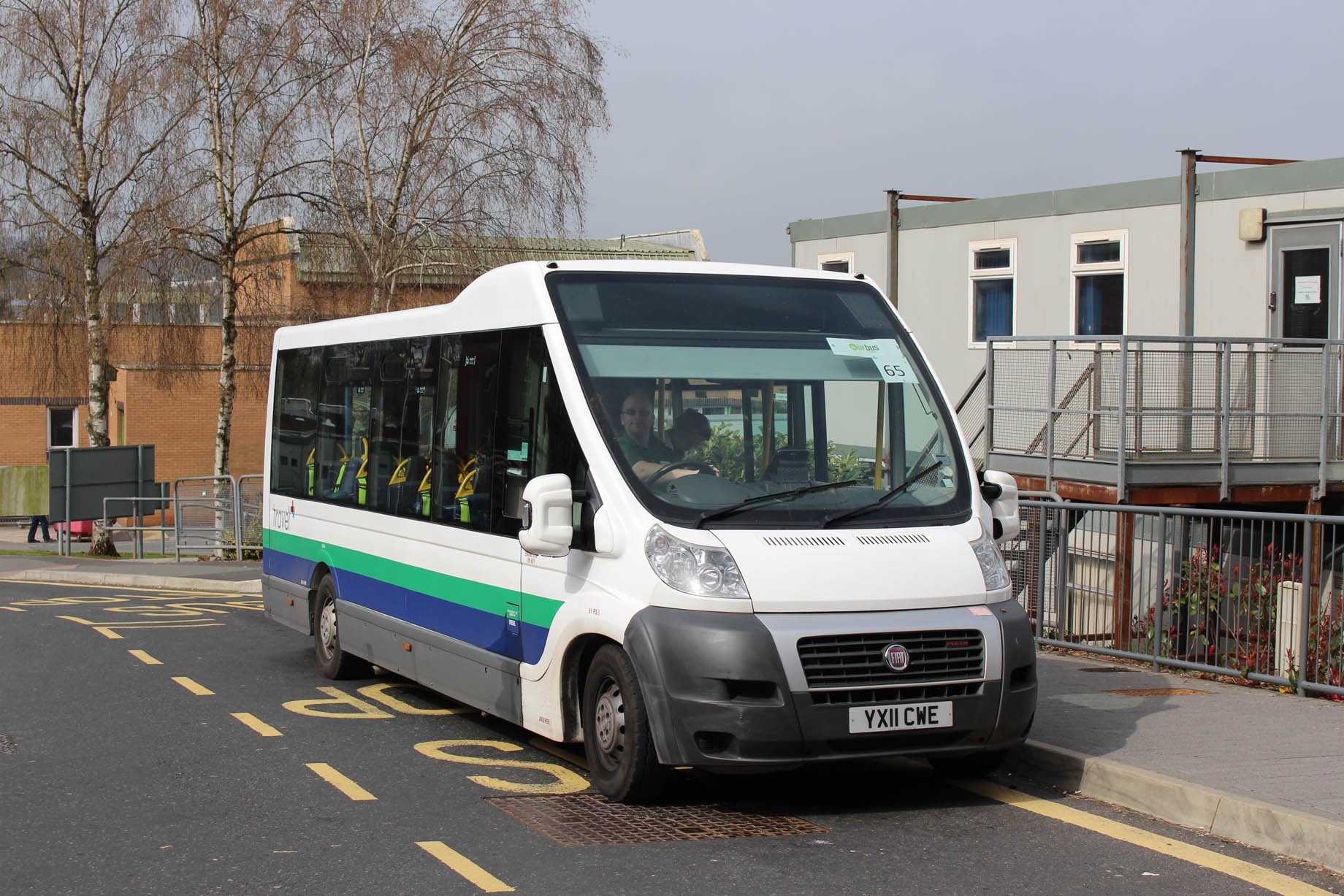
[622,409,651,416]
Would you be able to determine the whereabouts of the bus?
[261,262,1039,809]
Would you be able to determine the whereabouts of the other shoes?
[45,538,56,543]
[28,540,40,543]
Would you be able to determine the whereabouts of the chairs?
[305,435,507,529]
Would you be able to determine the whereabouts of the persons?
[27,515,56,543]
[619,389,721,485]
[666,407,712,455]
